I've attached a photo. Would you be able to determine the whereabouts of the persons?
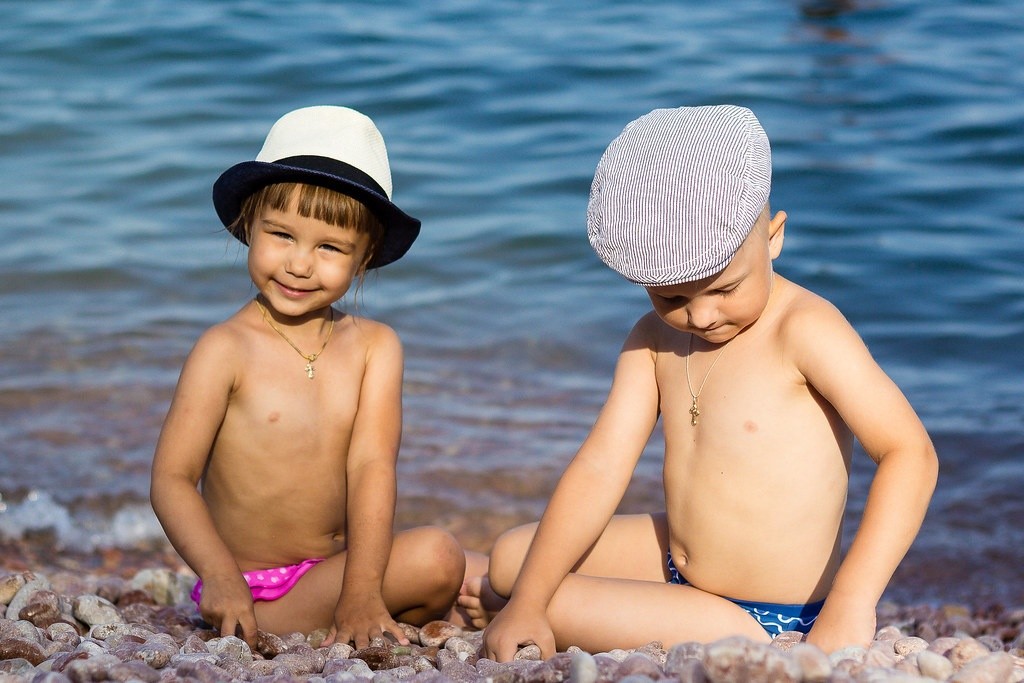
[455,103,939,665]
[147,103,467,652]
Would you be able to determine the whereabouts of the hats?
[213,105,422,270]
[586,105,773,287]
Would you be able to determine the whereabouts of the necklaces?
[684,334,730,428]
[254,298,336,381]
